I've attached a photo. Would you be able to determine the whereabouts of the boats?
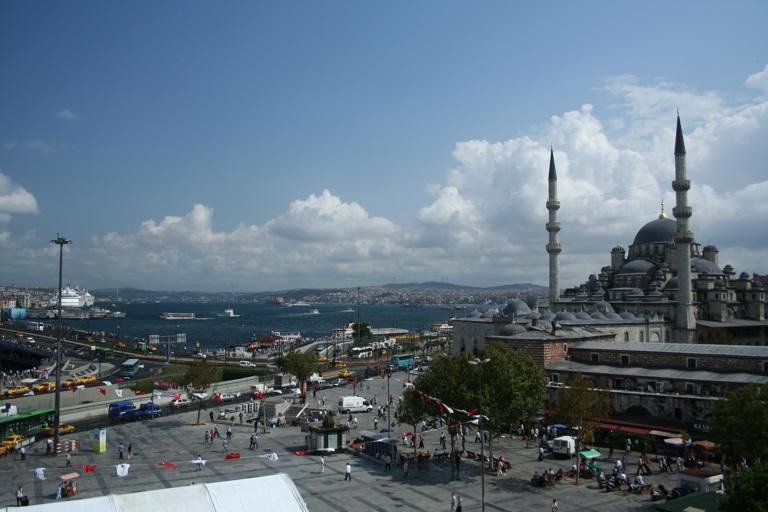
[305,308,321,315]
[217,309,242,318]
[341,307,355,312]
[160,312,214,321]
[281,300,311,307]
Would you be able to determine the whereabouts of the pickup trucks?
[131,403,162,420]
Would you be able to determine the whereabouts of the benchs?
[399,450,653,494]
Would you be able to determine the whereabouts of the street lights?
[25,292,28,312]
[381,362,394,438]
[356,285,362,346]
[116,323,121,341]
[50,230,73,456]
[467,357,492,512]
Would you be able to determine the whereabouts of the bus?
[26,322,44,332]
[0,408,56,445]
[352,346,373,360]
[119,358,142,377]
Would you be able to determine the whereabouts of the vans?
[108,400,136,420]
[252,384,273,396]
[552,436,578,458]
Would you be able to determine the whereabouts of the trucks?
[239,360,256,367]
[338,394,374,414]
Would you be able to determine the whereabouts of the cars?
[316,344,324,351]
[0,445,10,456]
[170,398,194,407]
[15,331,24,338]
[1,375,100,396]
[289,355,354,394]
[146,345,157,352]
[380,346,433,371]
[72,334,126,362]
[27,336,36,343]
[2,434,26,447]
[41,423,76,437]
[191,353,206,359]
[215,392,237,402]
[266,363,279,369]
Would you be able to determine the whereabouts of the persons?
[2,338,761,510]
